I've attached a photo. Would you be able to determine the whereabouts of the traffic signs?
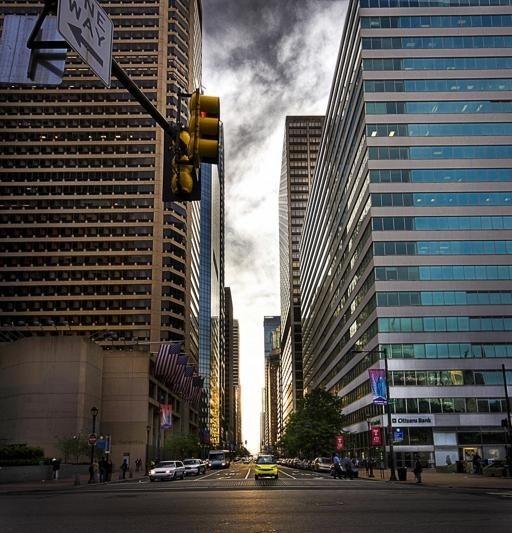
[58,0,114,88]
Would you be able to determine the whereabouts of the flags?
[154,342,205,403]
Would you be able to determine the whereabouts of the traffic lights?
[188,88,220,167]
[88,405,98,484]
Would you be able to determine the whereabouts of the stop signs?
[88,433,97,446]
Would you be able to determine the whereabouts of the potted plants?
[0,443,54,482]
[69,436,94,484]
[55,435,76,478]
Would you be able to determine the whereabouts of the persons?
[52,458,61,480]
[471,452,484,474]
[411,456,423,483]
[333,454,386,480]
[445,454,450,465]
[89,454,160,483]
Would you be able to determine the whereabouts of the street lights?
[144,425,151,476]
[350,349,398,482]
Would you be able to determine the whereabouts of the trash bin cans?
[73,471,80,486]
[397,467,408,481]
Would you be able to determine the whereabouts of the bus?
[208,450,230,469]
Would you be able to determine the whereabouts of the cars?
[254,453,279,480]
[280,456,358,477]
[148,459,207,482]
